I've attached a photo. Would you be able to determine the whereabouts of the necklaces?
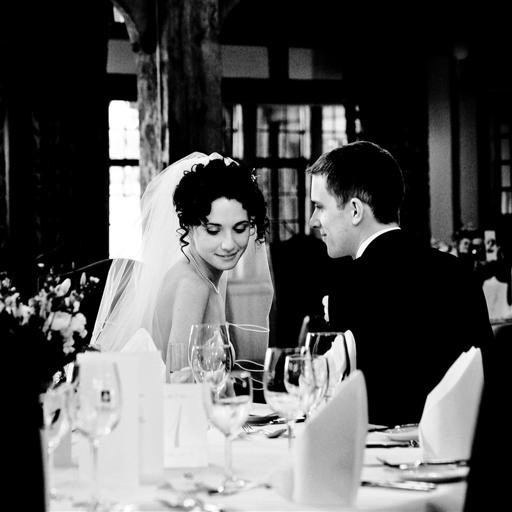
[185,249,220,294]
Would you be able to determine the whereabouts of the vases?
[0,397,46,512]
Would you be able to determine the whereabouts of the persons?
[307,141,497,439]
[151,159,270,405]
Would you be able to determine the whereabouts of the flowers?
[1,264,89,393]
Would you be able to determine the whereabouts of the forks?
[374,454,471,473]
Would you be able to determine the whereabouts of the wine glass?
[63,356,126,510]
[184,320,350,494]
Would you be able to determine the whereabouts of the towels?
[420,345,485,466]
[292,373,368,512]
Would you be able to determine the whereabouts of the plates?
[379,444,471,485]
